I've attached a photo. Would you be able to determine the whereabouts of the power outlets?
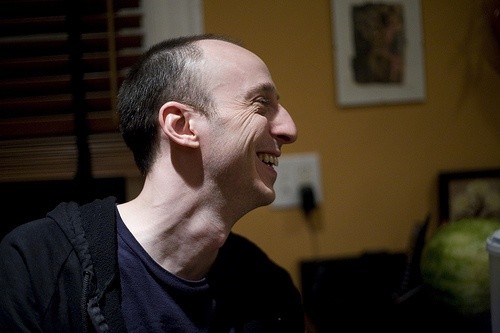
[269,150,325,211]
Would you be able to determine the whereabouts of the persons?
[0,34,306,333]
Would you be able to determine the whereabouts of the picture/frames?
[328,0,428,110]
